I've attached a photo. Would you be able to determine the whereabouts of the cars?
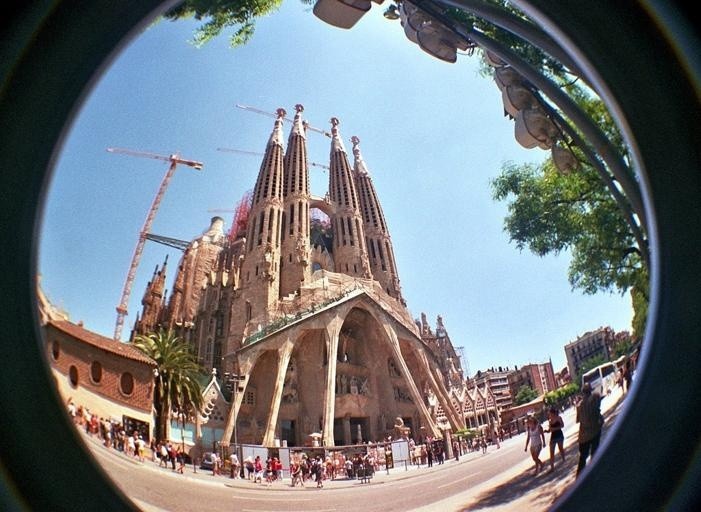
[171,452,192,465]
[199,452,216,470]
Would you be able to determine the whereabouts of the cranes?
[100,142,203,342]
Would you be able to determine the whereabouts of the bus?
[579,355,626,406]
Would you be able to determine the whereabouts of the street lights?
[223,370,247,455]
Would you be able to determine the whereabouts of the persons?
[67,345,641,489]
